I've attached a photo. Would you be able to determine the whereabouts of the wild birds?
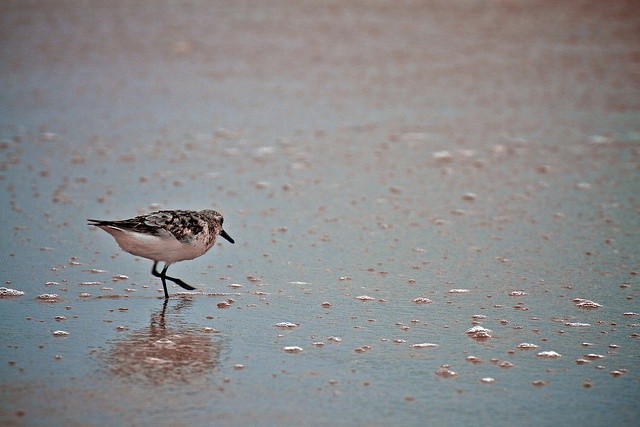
[85,207,235,298]
